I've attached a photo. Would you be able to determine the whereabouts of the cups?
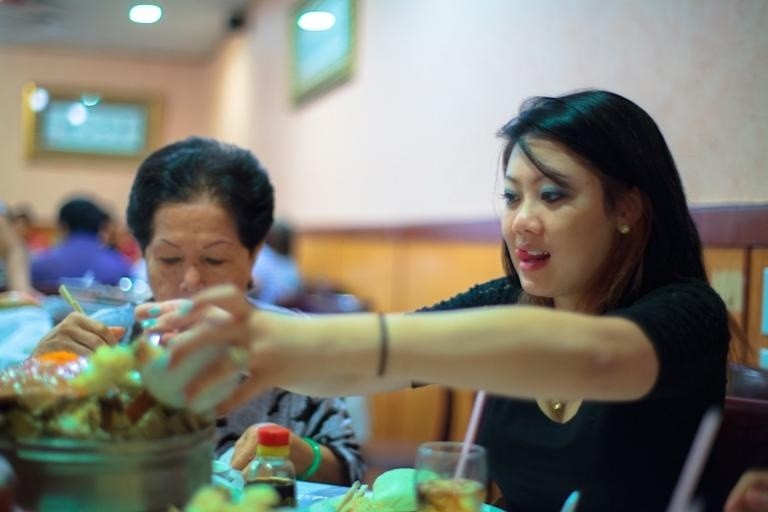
[413,442,487,512]
[371,465,440,498]
[140,327,243,415]
[210,458,232,481]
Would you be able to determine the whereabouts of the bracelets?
[297,437,323,480]
[373,309,389,374]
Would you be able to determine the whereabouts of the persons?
[2,199,301,305]
[724,466,767,512]
[30,134,368,487]
[130,86,732,512]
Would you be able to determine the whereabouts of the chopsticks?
[58,283,83,313]
[335,479,369,512]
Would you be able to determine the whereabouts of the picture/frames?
[20,80,166,170]
[285,1,356,110]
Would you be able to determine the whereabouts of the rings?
[228,346,240,363]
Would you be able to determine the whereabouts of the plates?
[227,468,245,493]
[309,494,344,511]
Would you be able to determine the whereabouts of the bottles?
[246,425,297,506]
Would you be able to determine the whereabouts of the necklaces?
[549,400,566,418]
